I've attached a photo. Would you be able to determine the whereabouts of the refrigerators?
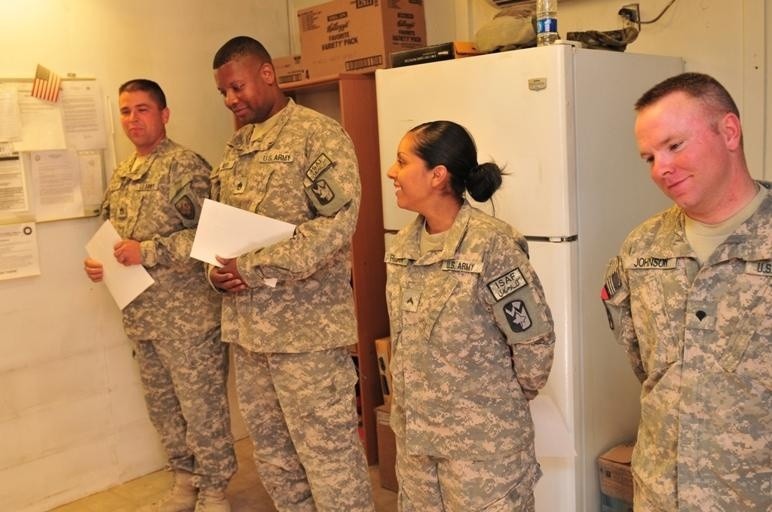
[375,45,689,512]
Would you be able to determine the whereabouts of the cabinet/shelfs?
[234,73,398,469]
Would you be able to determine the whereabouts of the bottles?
[535,0,559,47]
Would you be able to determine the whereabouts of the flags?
[31,65,62,101]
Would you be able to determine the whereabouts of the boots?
[150,467,198,512]
[194,488,232,512]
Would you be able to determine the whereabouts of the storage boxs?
[296,0,428,75]
[371,405,399,493]
[270,55,303,85]
[597,438,637,505]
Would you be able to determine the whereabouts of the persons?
[383,121,555,512]
[202,36,376,512]
[84,79,238,512]
[603,72,772,512]
